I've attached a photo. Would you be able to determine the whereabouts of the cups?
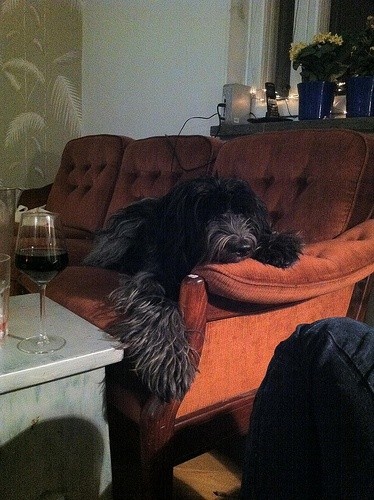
[0,253,12,346]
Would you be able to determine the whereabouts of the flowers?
[288,14,374,82]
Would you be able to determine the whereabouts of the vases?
[297,82,337,120]
[344,75,374,117]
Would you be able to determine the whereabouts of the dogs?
[79,157,312,414]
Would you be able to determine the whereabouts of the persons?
[243,318,374,500]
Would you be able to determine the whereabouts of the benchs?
[9,128,374,500]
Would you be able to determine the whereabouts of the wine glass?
[14,210,67,355]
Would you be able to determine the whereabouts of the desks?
[0,291,123,500]
